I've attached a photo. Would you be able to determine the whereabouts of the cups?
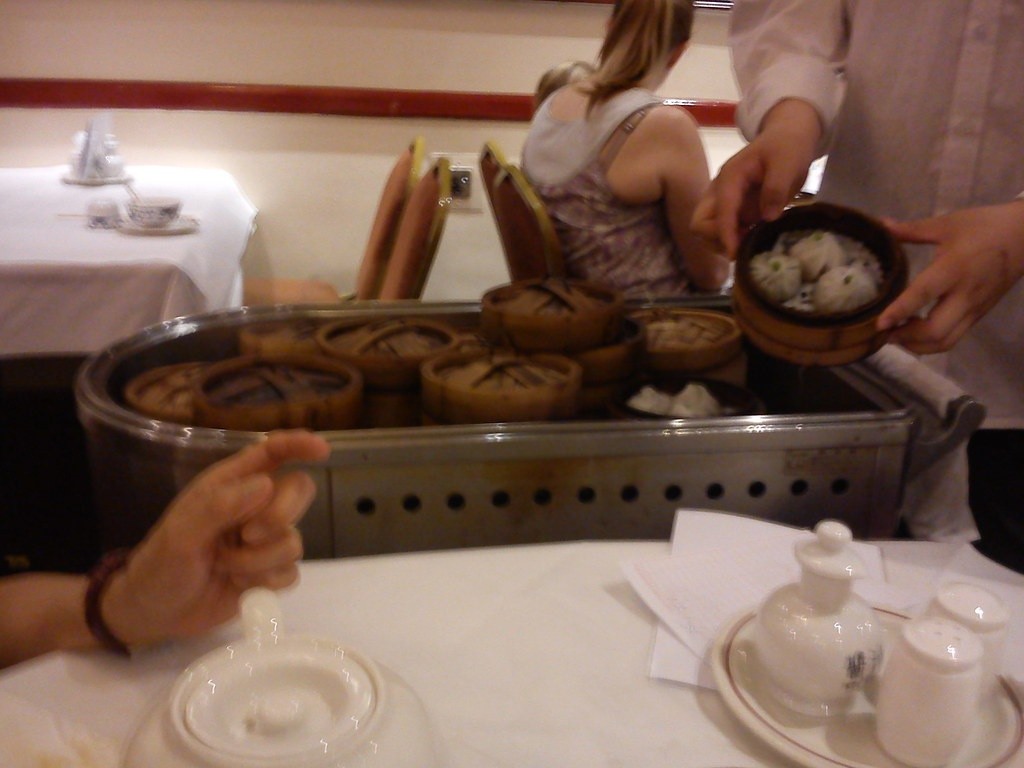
[875,578,1010,767]
[87,197,124,229]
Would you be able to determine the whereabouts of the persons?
[0,430,335,668]
[689,0,1024,573]
[522,0,729,301]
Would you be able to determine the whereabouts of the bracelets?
[83,549,132,657]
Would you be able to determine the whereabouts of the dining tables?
[0,536,1024,768]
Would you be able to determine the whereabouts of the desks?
[1,159,261,397]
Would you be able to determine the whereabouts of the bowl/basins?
[126,200,180,227]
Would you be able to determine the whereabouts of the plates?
[62,172,133,185]
[168,634,387,768]
[116,215,199,235]
[709,604,1024,768]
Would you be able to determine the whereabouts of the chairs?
[243,133,569,309]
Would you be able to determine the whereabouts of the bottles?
[759,520,882,717]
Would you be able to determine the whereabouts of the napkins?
[626,510,885,693]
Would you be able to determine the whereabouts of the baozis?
[746,227,876,316]
